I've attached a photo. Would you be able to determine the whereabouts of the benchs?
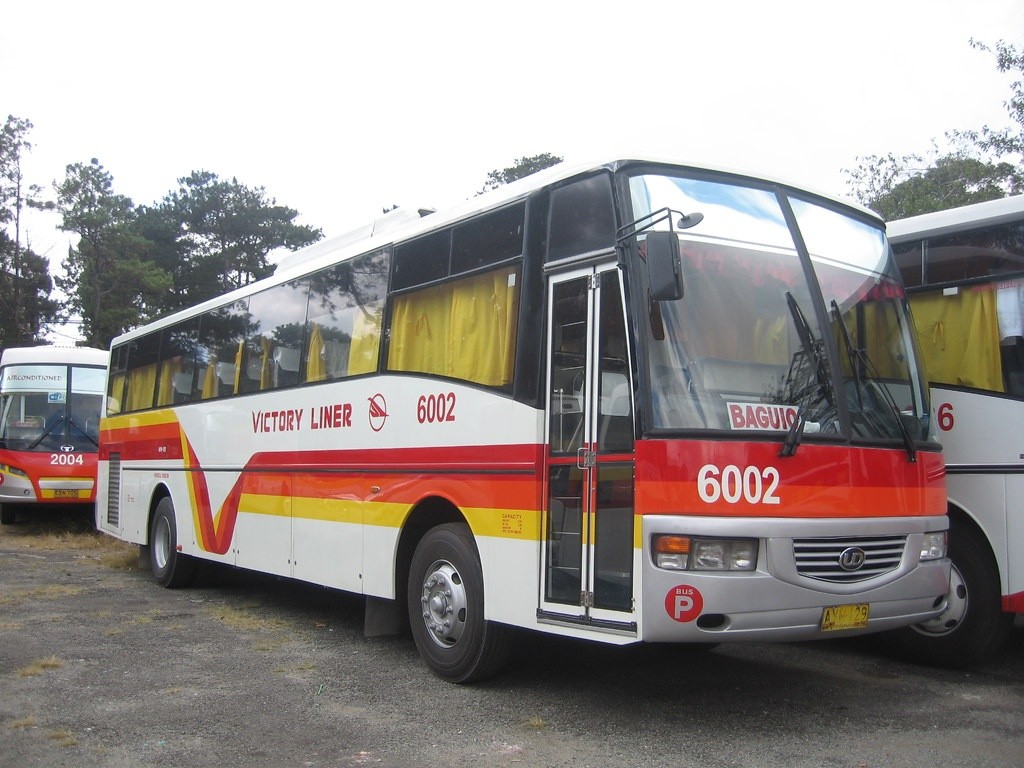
[25,416,46,429]
[84,417,99,434]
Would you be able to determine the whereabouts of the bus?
[95,150,950,685]
[0,344,111,524]
[885,193,1024,669]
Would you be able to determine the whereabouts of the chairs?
[215,361,236,396]
[174,374,191,404]
[323,339,350,378]
[242,358,273,391]
[272,346,300,388]
